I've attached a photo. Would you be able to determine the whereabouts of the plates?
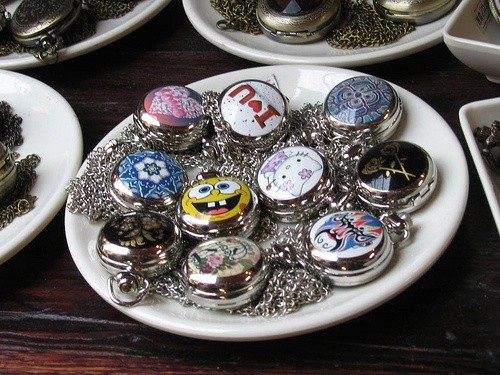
[182,0,462,67]
[0,0,171,69]
[0,69,84,265]
[459,97,500,235]
[65,64,469,341]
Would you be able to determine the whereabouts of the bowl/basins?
[444,0,500,84]
